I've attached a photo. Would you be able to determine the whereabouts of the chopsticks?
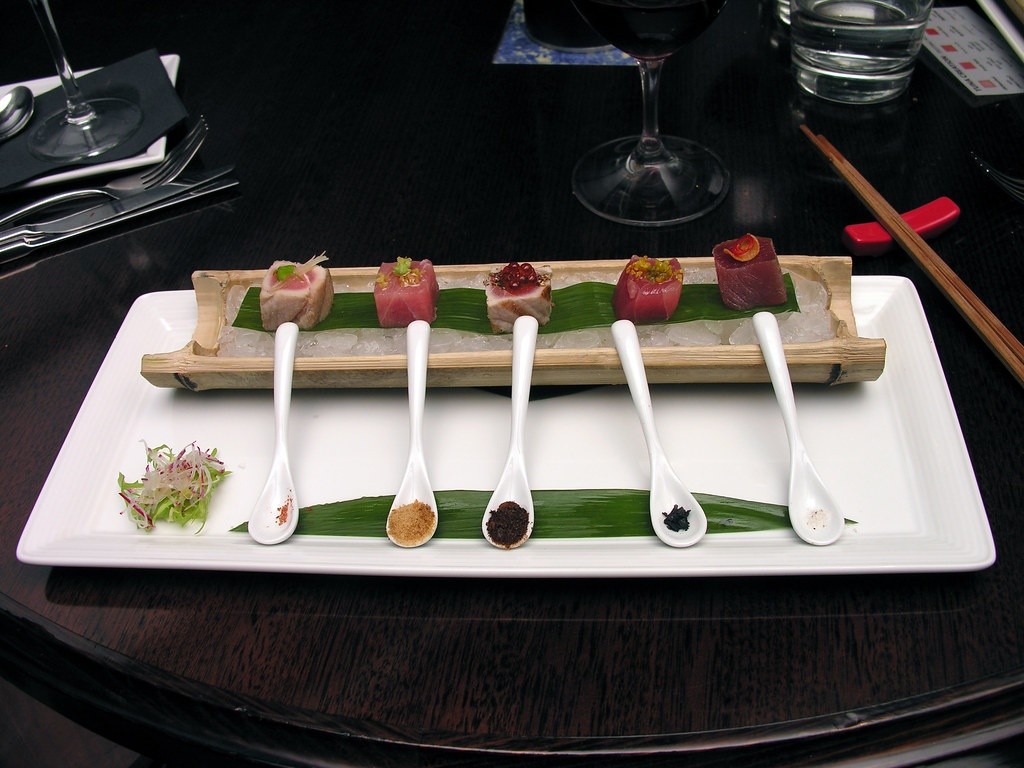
[798,123,1024,390]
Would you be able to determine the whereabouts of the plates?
[16,273,996,580]
[974,0,1024,64]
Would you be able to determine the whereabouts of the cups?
[788,0,936,108]
[773,0,790,25]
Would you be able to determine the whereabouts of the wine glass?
[565,0,734,229]
[29,0,144,167]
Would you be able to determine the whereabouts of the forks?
[0,112,211,227]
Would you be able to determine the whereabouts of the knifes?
[0,162,242,254]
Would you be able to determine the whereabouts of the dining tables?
[0,0,1023,768]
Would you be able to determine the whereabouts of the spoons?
[0,85,35,141]
[609,320,709,551]
[386,317,439,551]
[481,314,538,552]
[247,320,301,546]
